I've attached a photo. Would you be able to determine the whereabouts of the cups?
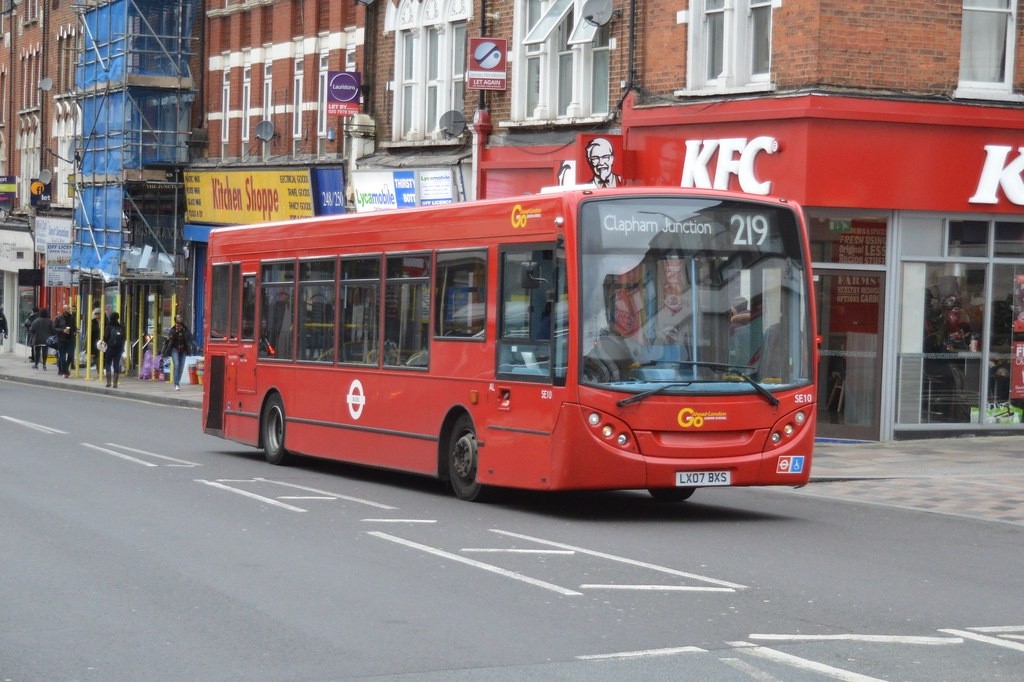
[66,327,70,334]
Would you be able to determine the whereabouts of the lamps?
[941,222,968,277]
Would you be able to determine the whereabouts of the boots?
[113,372,118,388]
[106,370,111,387]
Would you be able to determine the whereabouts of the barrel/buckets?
[188,357,204,385]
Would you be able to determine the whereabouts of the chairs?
[922,368,949,423]
[367,350,421,366]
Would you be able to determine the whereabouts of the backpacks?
[110,325,123,349]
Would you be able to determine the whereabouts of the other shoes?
[43,365,47,371]
[63,372,70,379]
[32,365,38,370]
[174,382,179,392]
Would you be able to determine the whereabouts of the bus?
[201,185,825,504]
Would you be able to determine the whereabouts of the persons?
[728,297,803,380]
[168,314,200,391]
[0,302,126,389]
[829,342,847,424]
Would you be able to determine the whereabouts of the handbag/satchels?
[46,335,59,350]
[161,341,169,356]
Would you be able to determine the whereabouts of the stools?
[825,372,845,411]
